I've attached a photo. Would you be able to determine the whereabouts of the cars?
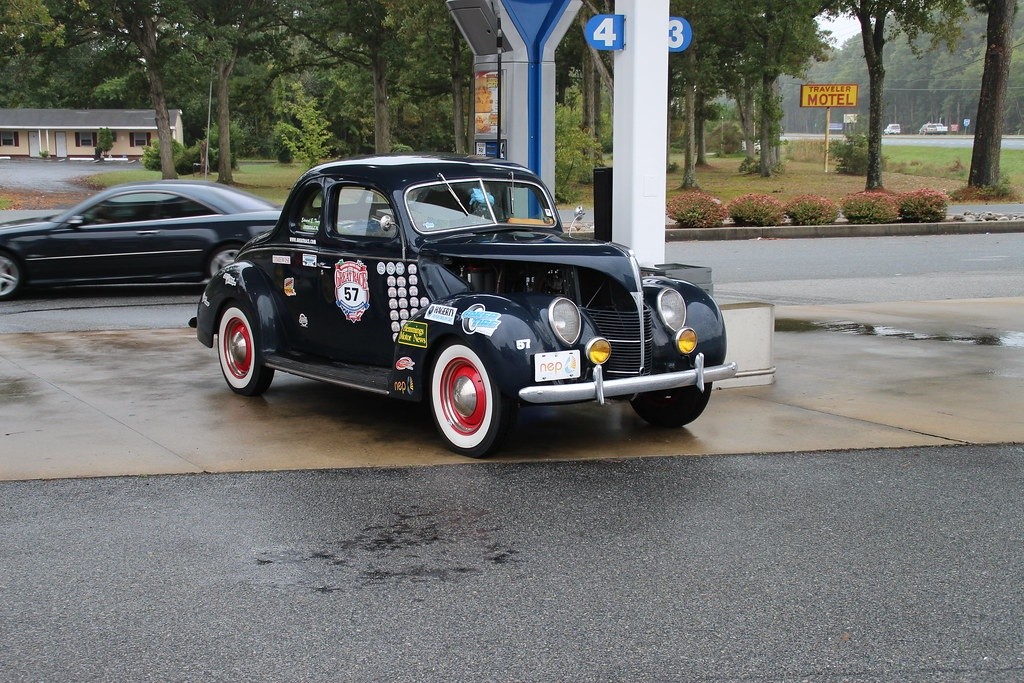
[0,179,284,301]
[188,154,735,456]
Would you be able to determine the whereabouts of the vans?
[920,125,937,135]
[884,124,900,135]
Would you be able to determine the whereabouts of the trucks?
[934,124,948,135]
